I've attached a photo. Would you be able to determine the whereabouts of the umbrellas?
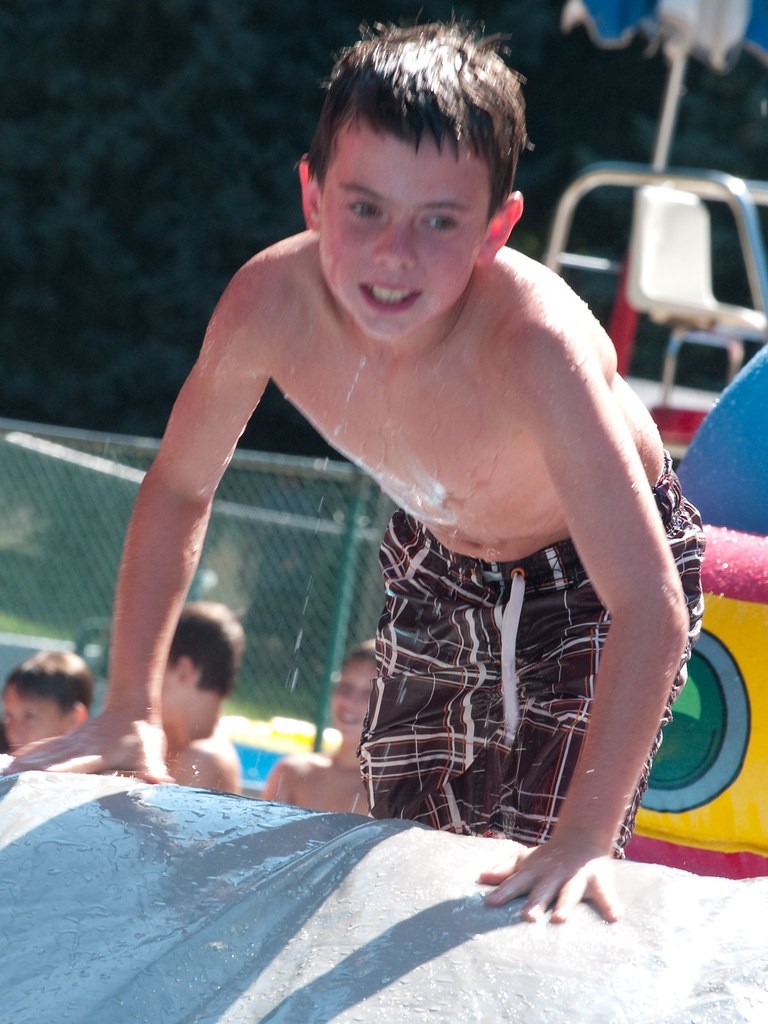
[559,0,768,170]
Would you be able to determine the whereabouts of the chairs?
[624,186,768,409]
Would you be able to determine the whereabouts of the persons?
[0,26,704,924]
[160,602,241,792]
[3,651,94,752]
[263,639,378,817]
